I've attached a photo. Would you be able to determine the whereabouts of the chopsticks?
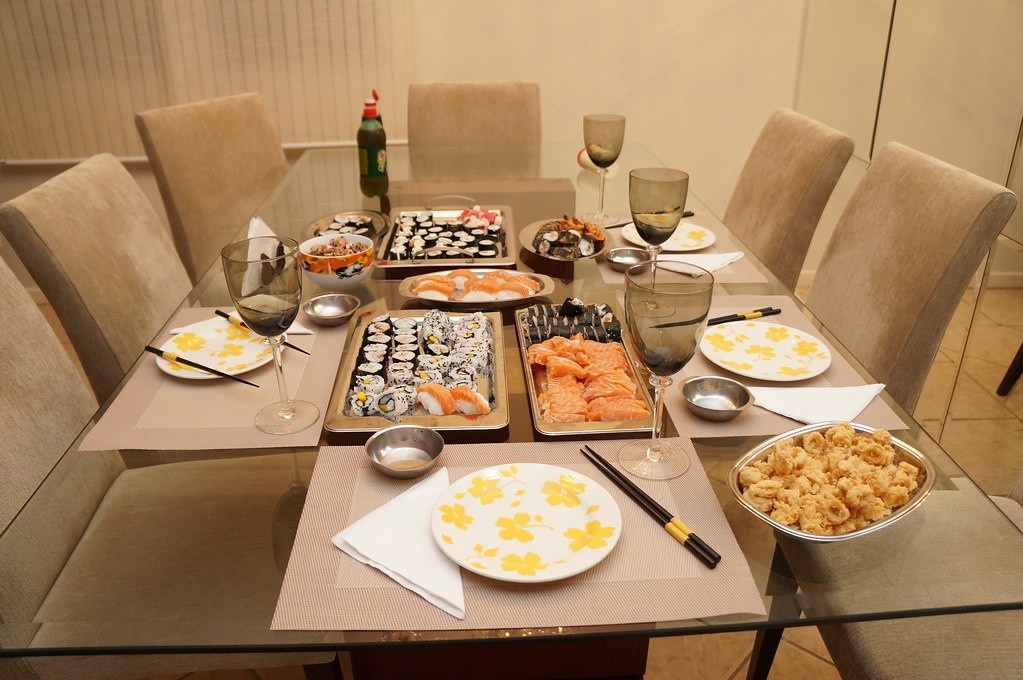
[144,309,311,388]
[646,306,781,329]
[604,211,694,230]
[580,444,721,569]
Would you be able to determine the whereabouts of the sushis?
[411,268,541,301]
[390,206,503,257]
[316,214,373,237]
[524,297,622,345]
[532,216,605,259]
[344,309,493,414]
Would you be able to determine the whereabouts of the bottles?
[356,88,389,199]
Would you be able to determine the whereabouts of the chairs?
[134,95,291,281]
[750,485,1023,680]
[407,83,542,151]
[722,107,854,294]
[0,152,195,395]
[793,143,1017,409]
[0,268,338,678]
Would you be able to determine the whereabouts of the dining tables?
[3,144,1023,659]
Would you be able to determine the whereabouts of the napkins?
[169,309,313,337]
[750,382,885,426]
[656,251,746,278]
[240,215,293,295]
[330,468,467,622]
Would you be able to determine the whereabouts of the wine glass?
[628,167,690,319]
[220,236,320,435]
[583,113,627,225]
[617,260,715,480]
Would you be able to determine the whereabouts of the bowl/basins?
[363,424,445,479]
[301,294,361,327]
[726,422,937,545]
[677,376,756,422]
[604,248,651,275]
[297,233,375,290]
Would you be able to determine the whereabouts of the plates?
[398,269,556,310]
[620,222,715,251]
[299,210,390,243]
[431,464,622,583]
[156,318,287,380]
[696,321,831,382]
[519,219,614,262]
